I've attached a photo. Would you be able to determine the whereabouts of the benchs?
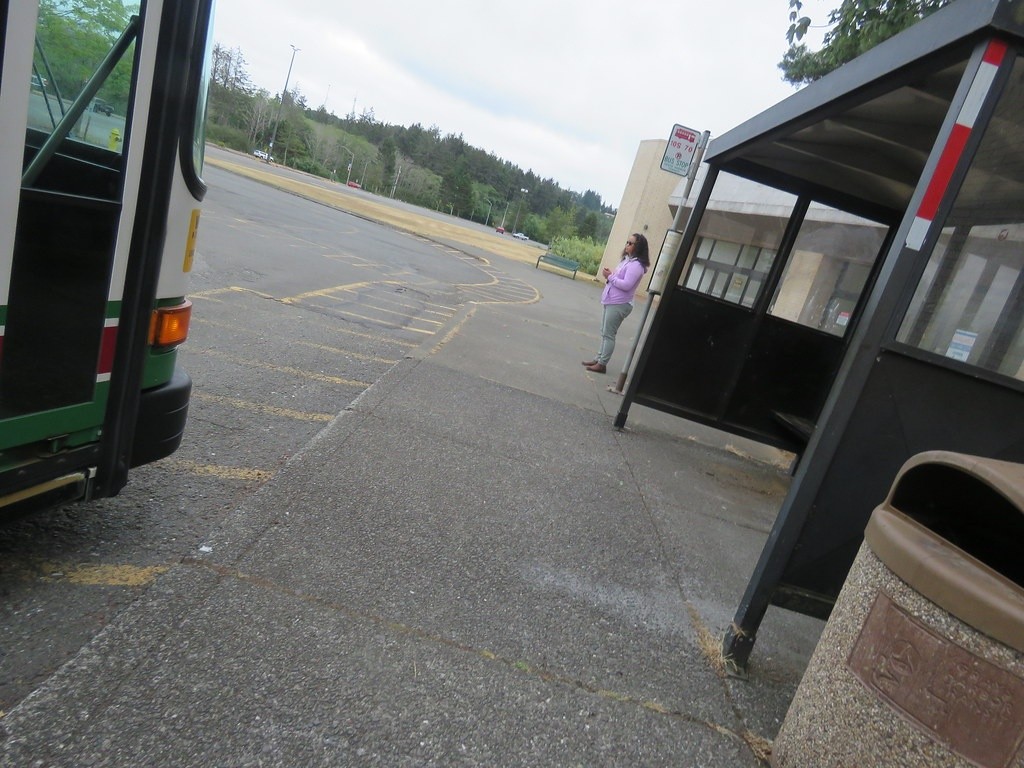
[535,252,579,280]
[771,409,817,448]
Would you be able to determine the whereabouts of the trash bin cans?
[772,450,1023,768]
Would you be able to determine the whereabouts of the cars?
[496,227,505,235]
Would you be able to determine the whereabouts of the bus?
[0,1,213,526]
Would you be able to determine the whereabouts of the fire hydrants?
[107,129,122,151]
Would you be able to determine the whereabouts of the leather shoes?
[583,360,598,366]
[586,363,606,374]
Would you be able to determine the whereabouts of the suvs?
[94,97,114,117]
[254,150,274,163]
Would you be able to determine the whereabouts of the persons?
[581,233,650,374]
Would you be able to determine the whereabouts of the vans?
[31,75,48,93]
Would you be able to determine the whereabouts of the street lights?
[511,188,529,237]
[266,44,301,165]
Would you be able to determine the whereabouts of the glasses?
[627,241,635,245]
